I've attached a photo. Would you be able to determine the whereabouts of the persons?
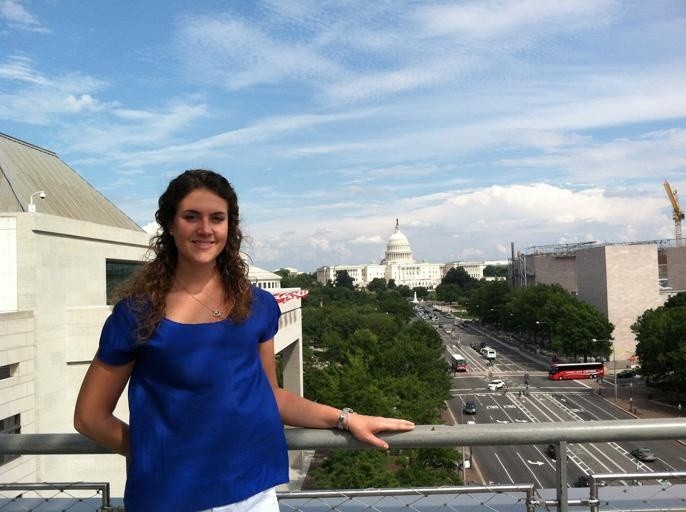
[74,167,414,512]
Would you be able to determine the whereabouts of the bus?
[451,355,466,372]
[549,361,604,380]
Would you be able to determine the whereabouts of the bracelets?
[336,408,357,434]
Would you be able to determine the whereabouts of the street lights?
[593,339,617,400]
[491,308,552,353]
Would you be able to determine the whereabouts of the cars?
[489,380,504,391]
[580,474,593,487]
[633,447,657,462]
[549,446,569,462]
[439,322,450,334]
[616,370,636,379]
[453,321,464,329]
[465,400,476,414]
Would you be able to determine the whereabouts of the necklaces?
[173,277,222,317]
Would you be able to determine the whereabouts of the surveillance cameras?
[40,192,46,200]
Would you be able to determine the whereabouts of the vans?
[480,347,497,359]
